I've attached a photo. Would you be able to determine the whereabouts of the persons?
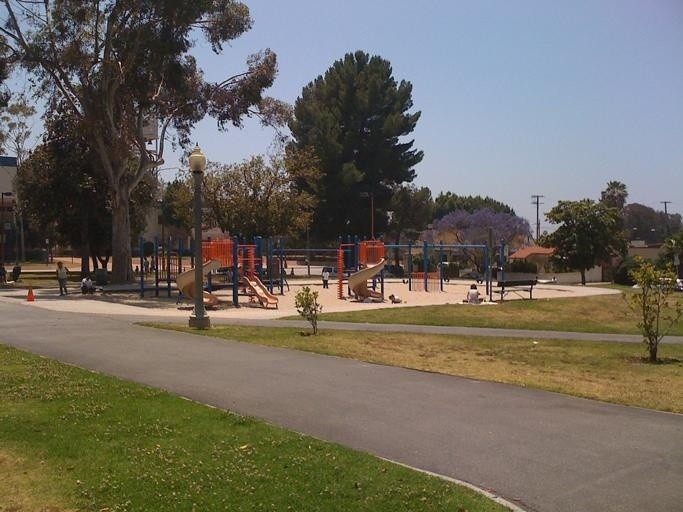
[80,275,93,296]
[0,263,7,284]
[389,294,403,304]
[135,256,156,273]
[466,283,481,304]
[56,261,72,296]
[226,268,233,283]
[290,267,294,278]
[322,268,329,289]
[12,261,21,282]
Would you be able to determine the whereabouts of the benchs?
[492,272,537,302]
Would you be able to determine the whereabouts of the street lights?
[189,144,211,327]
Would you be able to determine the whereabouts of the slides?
[243,276,279,304]
[348,258,385,302]
[176,260,218,305]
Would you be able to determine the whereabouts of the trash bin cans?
[95,268,108,286]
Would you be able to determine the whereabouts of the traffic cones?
[27,282,36,301]
